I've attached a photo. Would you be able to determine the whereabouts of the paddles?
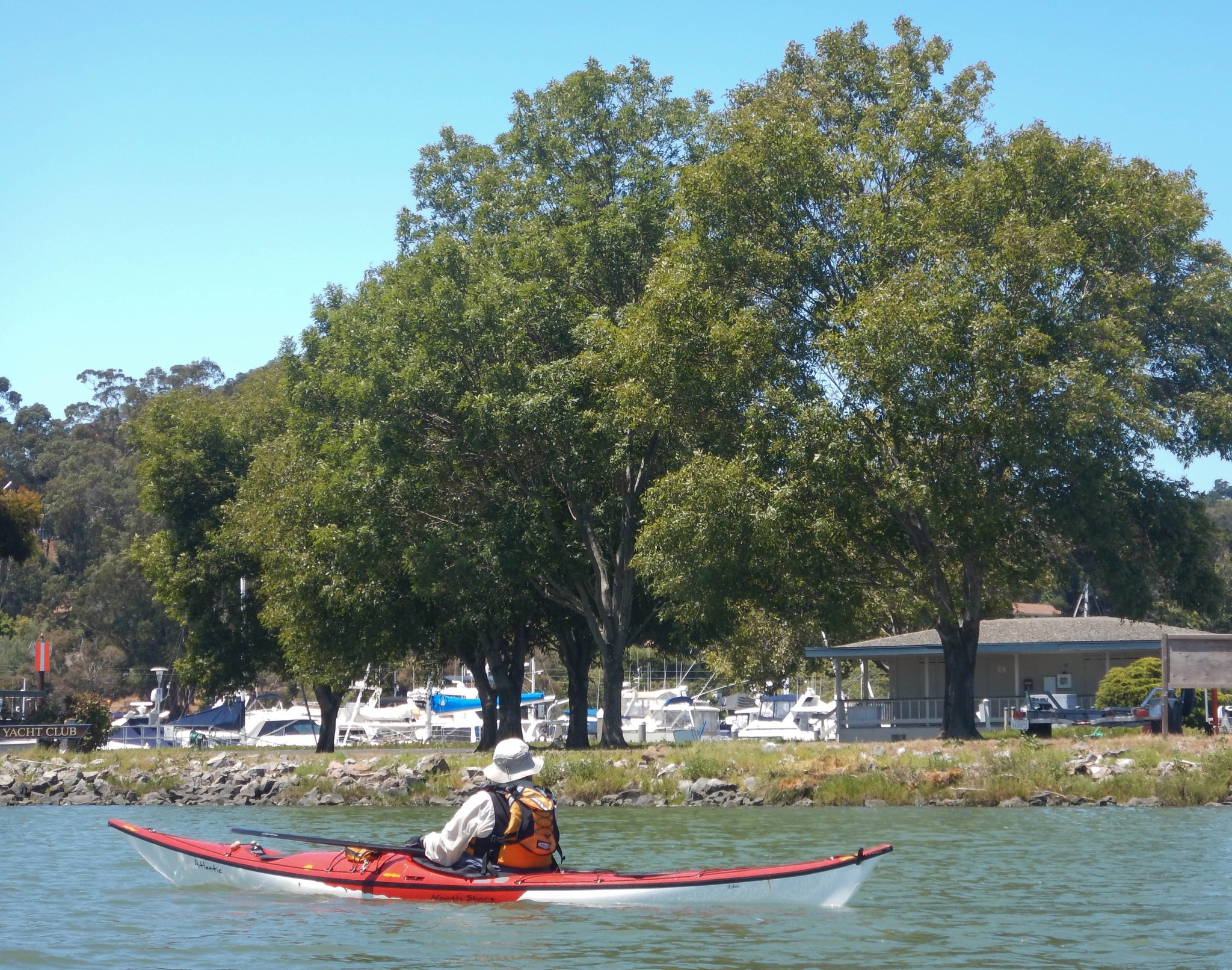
[229,825,428,859]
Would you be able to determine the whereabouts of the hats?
[483,738,544,783]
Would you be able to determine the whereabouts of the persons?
[404,738,560,873]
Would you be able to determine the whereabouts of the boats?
[106,842,894,915]
[89,652,891,758]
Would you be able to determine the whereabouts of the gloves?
[405,835,423,848]
